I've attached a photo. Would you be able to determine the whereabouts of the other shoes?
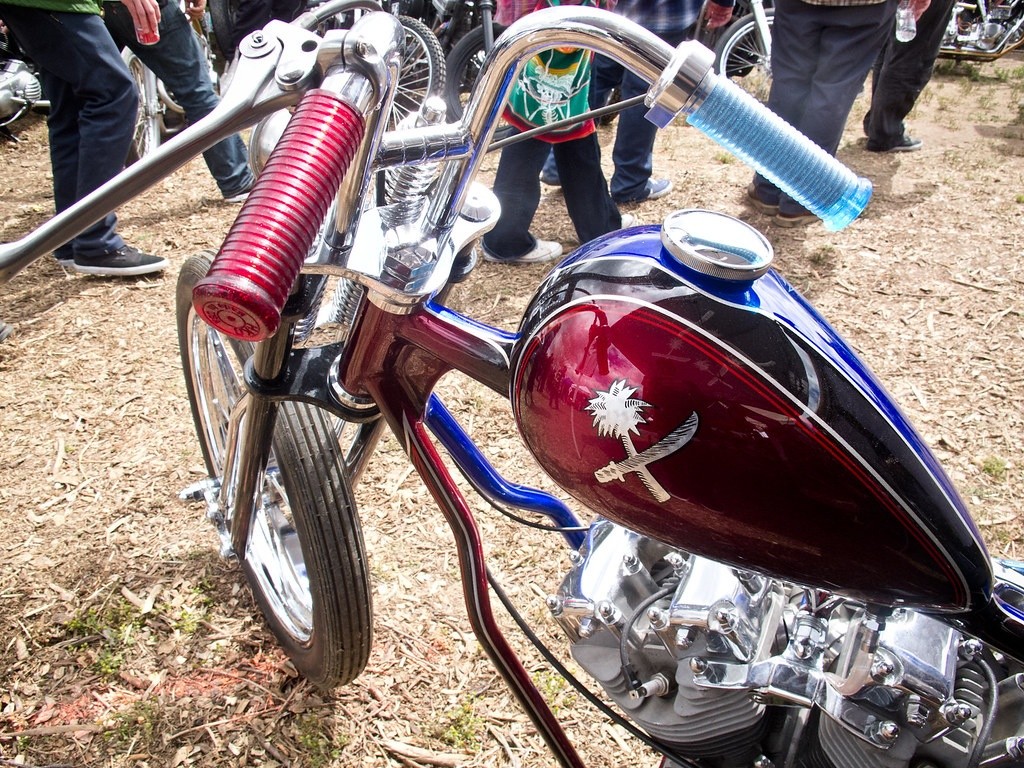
[482,240,563,263]
[890,136,922,152]
[540,176,561,190]
[51,244,169,277]
[616,180,670,203]
[772,212,821,228]
[223,190,251,202]
[748,182,779,215]
[621,213,634,229]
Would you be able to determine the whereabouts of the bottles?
[896,0,915,42]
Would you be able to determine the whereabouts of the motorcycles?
[0,0,1024,768]
[0,0,1024,172]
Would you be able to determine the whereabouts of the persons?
[104,0,255,202]
[0,0,170,275]
[482,0,636,264]
[539,0,736,205]
[863,0,957,155]
[748,0,931,228]
